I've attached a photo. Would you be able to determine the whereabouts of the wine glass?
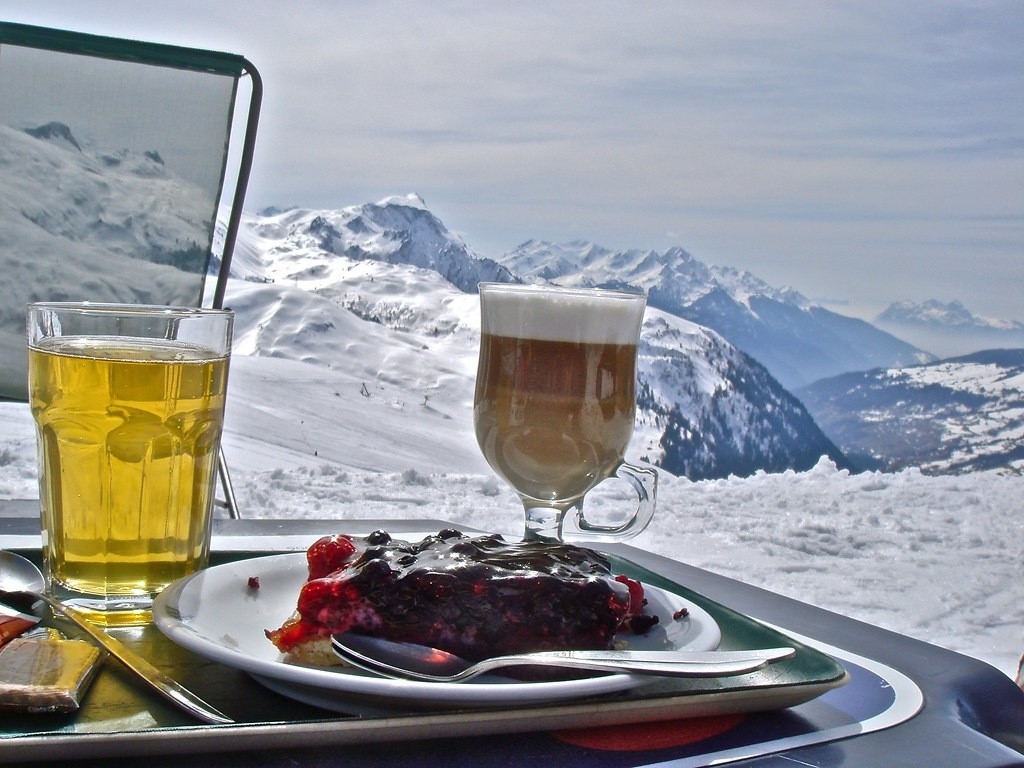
[472,281,659,547]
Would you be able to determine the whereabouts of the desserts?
[262,529,660,668]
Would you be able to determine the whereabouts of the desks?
[0,520,1024,768]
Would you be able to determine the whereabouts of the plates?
[151,551,722,701]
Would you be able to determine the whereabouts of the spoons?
[331,632,795,684]
[0,549,235,724]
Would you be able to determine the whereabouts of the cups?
[28,301,234,627]
